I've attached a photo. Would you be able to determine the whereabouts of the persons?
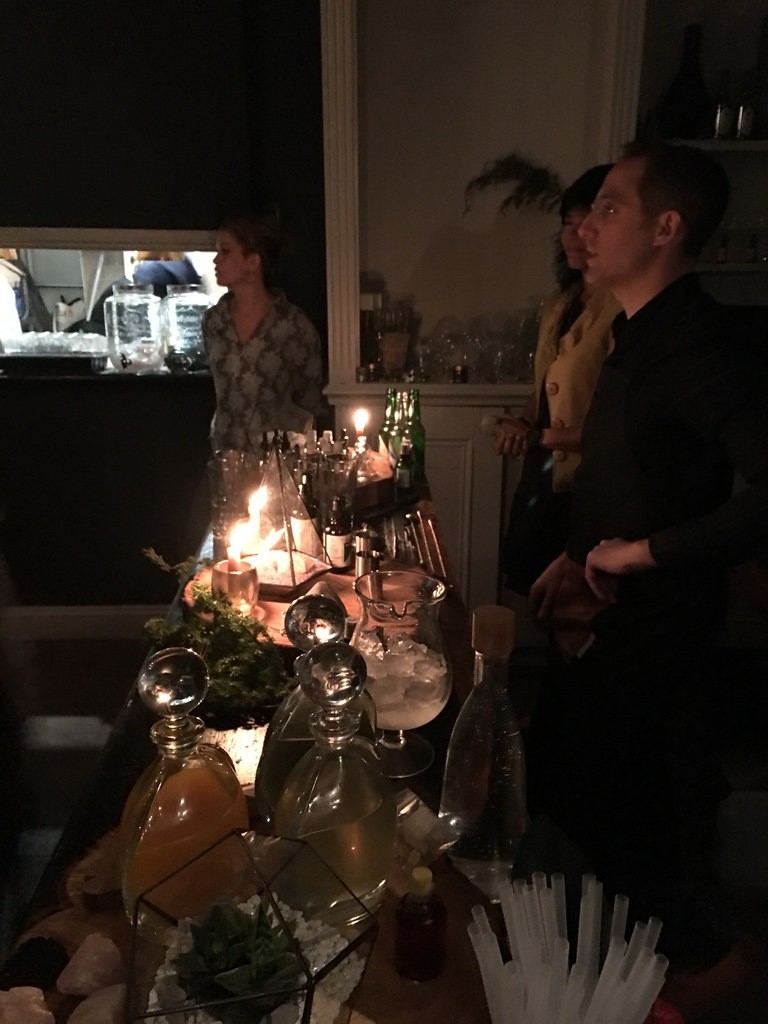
[492,164,626,721]
[520,136,768,953]
[201,219,326,462]
[90,251,185,325]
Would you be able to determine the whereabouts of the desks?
[0,475,529,1024]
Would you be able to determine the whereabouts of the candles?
[351,407,372,441]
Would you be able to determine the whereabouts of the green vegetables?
[167,899,307,1024]
[146,584,297,711]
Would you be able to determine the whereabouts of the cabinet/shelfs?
[319,0,768,612]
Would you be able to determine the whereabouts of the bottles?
[157,283,214,371]
[117,647,253,950]
[440,606,530,887]
[218,425,373,571]
[251,598,377,838]
[379,387,426,508]
[103,283,163,374]
[272,640,397,937]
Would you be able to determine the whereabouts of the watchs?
[525,426,542,446]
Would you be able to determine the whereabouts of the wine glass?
[347,569,452,779]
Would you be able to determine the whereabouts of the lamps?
[223,429,332,602]
